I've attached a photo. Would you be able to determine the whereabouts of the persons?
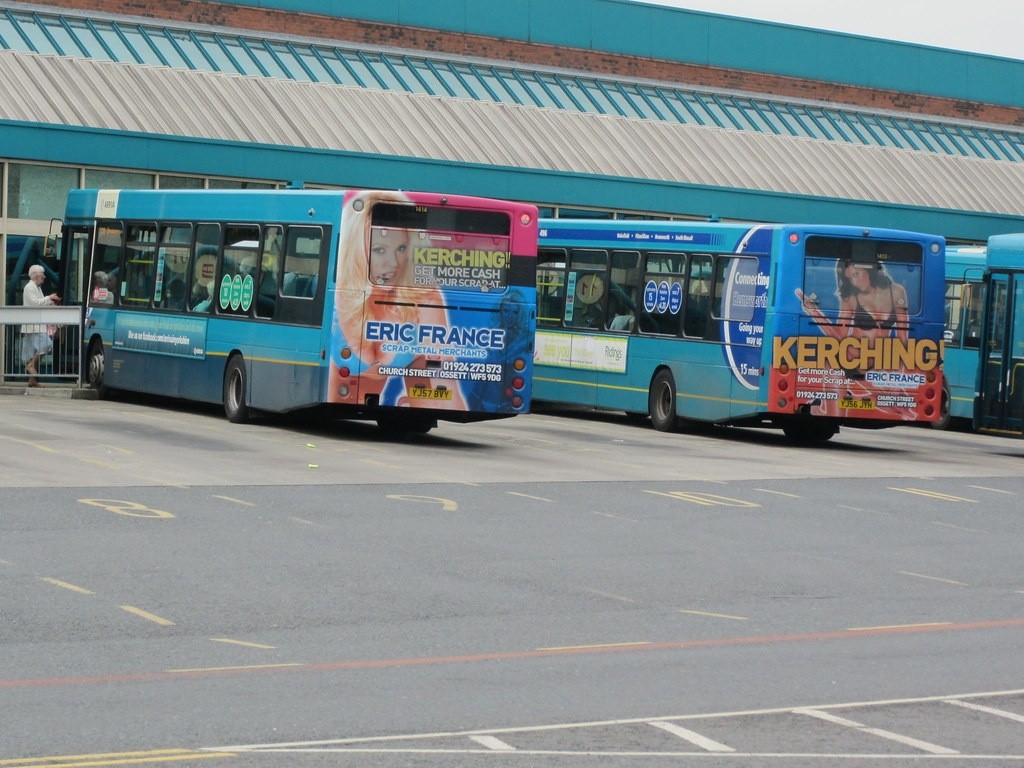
[93,248,320,321]
[796,242,918,421]
[582,283,720,339]
[328,189,535,415]
[20,263,62,387]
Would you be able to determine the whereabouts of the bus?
[40,187,539,438]
[959,233,1024,438]
[528,218,947,444]
[929,243,1024,430]
